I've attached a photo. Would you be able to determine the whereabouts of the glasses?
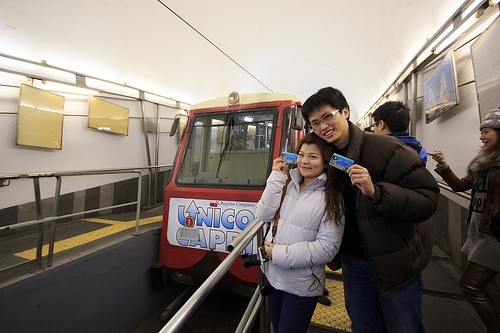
[311,109,338,129]
[370,122,379,131]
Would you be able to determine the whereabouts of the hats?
[480,104,500,130]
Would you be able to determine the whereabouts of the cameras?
[240,247,269,269]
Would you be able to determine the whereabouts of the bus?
[158,91,313,285]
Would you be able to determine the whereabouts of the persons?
[371,100,427,165]
[256,132,345,333]
[301,86,440,333]
[427,104,500,333]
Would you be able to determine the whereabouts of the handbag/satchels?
[259,269,270,296]
